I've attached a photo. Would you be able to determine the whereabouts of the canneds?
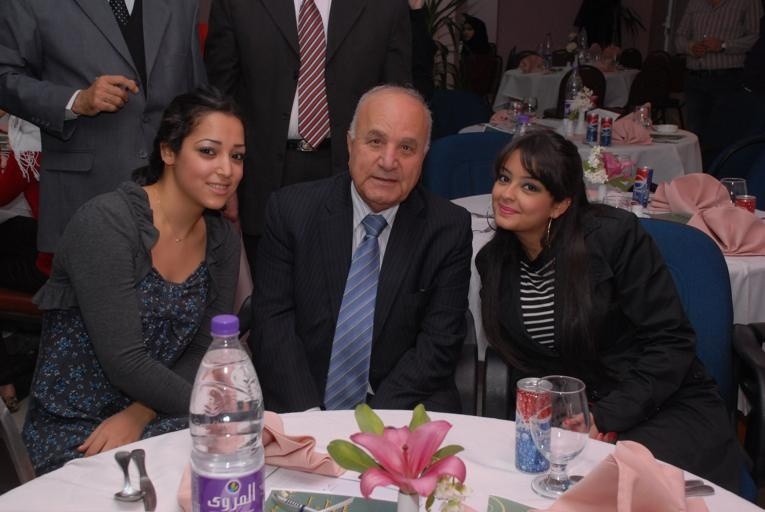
[632,166,653,208]
[586,112,600,143]
[735,195,756,213]
[514,377,554,475]
[600,116,612,146]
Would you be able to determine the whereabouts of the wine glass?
[531,375,592,498]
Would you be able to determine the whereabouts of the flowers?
[325,404,473,508]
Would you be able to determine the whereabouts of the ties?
[324,214,388,410]
[109,0,129,27]
[297,0,330,149]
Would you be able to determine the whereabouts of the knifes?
[130,448,158,512]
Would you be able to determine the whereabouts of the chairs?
[425,129,508,201]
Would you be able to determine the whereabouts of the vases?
[396,491,419,511]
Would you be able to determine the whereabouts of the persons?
[2,1,508,296]
[673,2,764,156]
[247,81,474,415]
[21,85,249,478]
[476,126,744,496]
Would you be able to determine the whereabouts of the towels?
[175,406,345,511]
[540,439,710,511]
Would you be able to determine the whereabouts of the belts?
[287,138,329,151]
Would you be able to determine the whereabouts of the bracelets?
[719,39,725,55]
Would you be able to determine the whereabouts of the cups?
[654,123,679,133]
[598,149,634,212]
[718,178,748,205]
[507,95,540,141]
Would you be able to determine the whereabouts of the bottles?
[563,69,580,132]
[189,315,265,512]
[587,112,597,142]
[541,32,592,77]
[601,117,612,145]
[639,105,650,131]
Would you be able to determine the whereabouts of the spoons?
[112,449,145,501]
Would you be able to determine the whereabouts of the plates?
[650,131,687,138]
